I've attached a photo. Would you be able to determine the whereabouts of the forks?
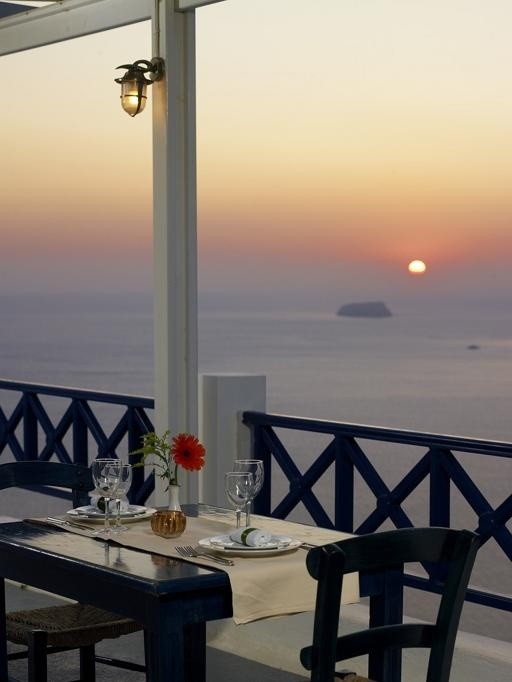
[176,545,234,567]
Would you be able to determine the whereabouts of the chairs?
[0,462,161,681]
[299,529,486,681]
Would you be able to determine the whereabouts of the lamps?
[114,57,164,117]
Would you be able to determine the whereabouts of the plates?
[68,505,158,524]
[197,535,302,557]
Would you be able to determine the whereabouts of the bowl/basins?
[152,510,186,539]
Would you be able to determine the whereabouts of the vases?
[165,485,184,510]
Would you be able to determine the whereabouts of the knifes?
[48,517,95,531]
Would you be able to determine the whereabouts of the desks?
[0,505,361,682]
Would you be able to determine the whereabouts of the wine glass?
[92,458,132,533]
[225,459,264,530]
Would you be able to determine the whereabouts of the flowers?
[130,429,206,486]
